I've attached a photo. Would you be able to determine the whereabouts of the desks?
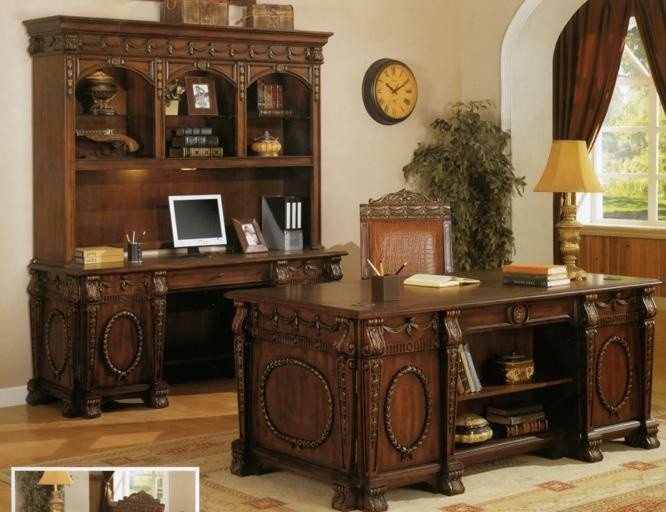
[223,264,664,512]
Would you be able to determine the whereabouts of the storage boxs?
[158,2,294,32]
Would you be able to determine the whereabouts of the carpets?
[0,424,665,512]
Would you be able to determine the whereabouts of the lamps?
[532,138,605,283]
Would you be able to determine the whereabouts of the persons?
[243,226,259,246]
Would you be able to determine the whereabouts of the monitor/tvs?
[168,194,228,259]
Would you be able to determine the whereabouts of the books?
[482,401,550,439]
[456,342,482,395]
[402,272,481,289]
[501,262,571,287]
[167,126,225,157]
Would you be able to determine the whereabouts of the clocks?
[361,57,419,126]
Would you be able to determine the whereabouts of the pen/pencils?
[125,230,145,243]
[367,259,408,276]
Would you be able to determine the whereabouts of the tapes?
[588,273,604,282]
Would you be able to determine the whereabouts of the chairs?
[358,189,453,278]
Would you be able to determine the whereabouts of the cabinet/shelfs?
[20,15,348,421]
